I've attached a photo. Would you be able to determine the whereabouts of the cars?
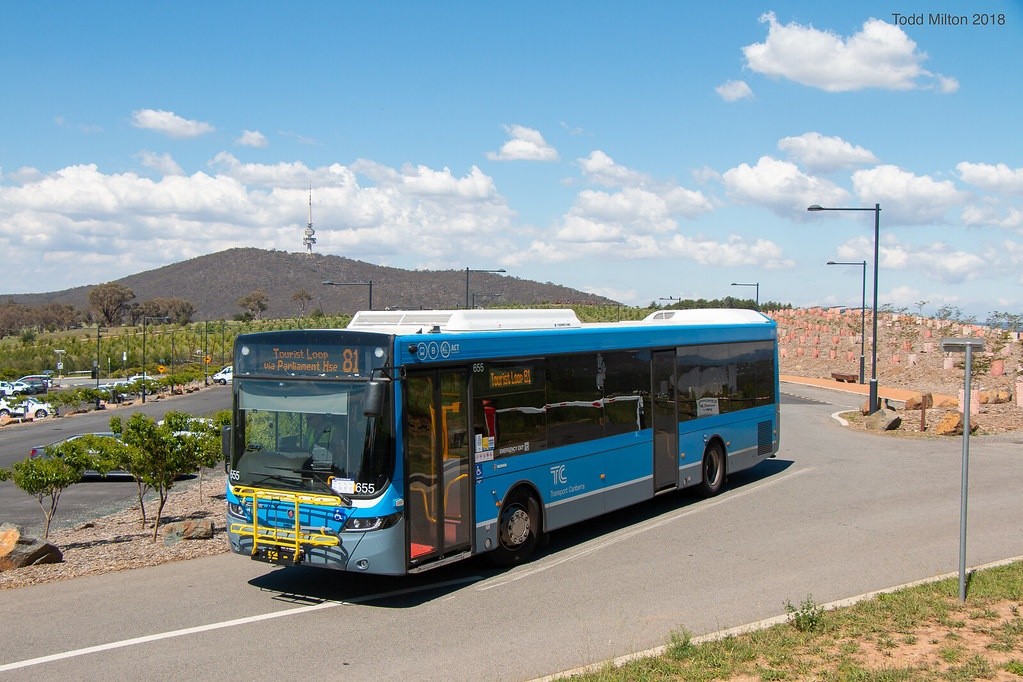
[1,373,200,419]
[29,417,220,482]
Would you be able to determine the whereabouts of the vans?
[212,365,235,386]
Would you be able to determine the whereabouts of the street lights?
[731,282,760,310]
[92,312,226,410]
[464,267,508,311]
[940,339,985,600]
[323,279,374,311]
[808,203,878,415]
[827,260,866,386]
[472,293,501,309]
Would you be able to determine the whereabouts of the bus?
[221,309,780,576]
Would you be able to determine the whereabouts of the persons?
[302,413,345,460]
